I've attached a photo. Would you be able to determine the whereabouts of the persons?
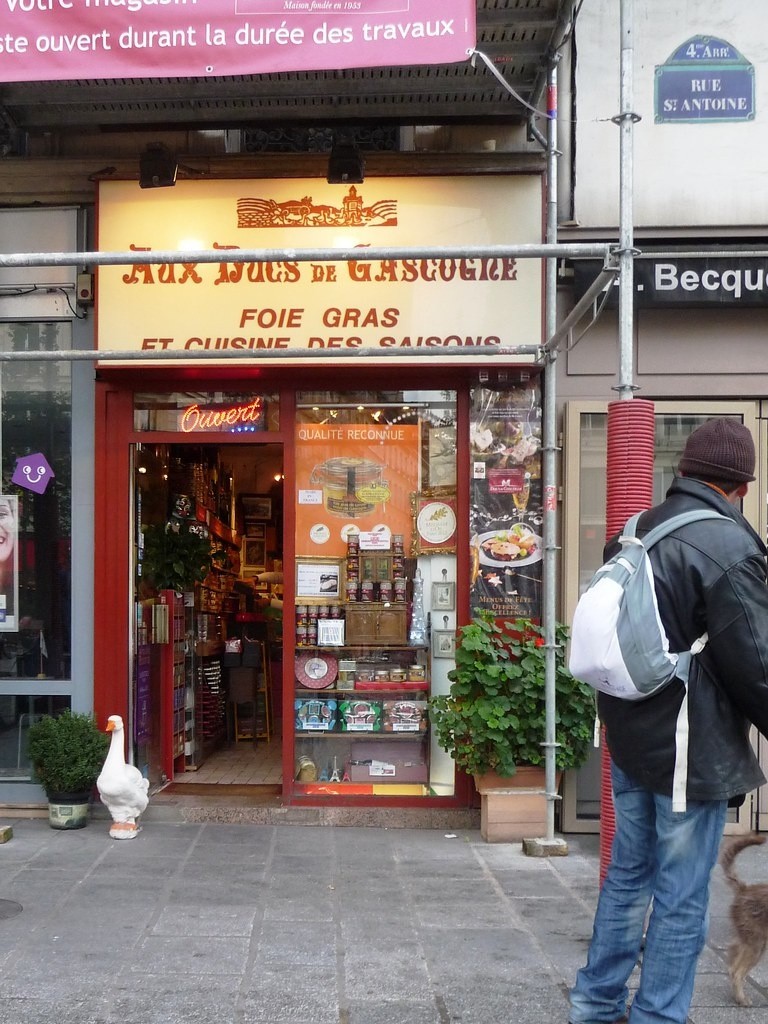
[567,416,768,1024]
[0,498,14,589]
[238,571,284,611]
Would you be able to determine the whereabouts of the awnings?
[0,0,575,148]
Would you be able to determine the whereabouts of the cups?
[470,531,478,589]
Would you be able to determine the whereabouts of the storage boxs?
[348,741,429,781]
[344,601,408,646]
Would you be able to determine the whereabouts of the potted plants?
[26,707,114,829]
[426,613,601,846]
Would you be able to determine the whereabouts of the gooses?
[96,714,149,826]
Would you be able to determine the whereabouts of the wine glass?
[512,474,529,530]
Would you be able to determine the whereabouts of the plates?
[470,443,506,455]
[478,530,543,567]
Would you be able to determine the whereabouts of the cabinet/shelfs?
[292,643,429,737]
[158,589,187,784]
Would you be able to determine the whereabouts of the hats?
[677,417,756,483]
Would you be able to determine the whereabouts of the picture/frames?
[295,555,347,606]
[432,580,455,611]
[431,628,456,659]
[409,484,457,557]
[239,492,274,590]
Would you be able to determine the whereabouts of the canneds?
[296,534,406,647]
[310,457,387,519]
[374,665,425,682]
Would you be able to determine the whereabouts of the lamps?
[138,140,181,188]
[328,140,365,184]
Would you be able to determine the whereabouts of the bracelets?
[252,575,259,582]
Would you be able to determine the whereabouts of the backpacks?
[568,503,730,703]
